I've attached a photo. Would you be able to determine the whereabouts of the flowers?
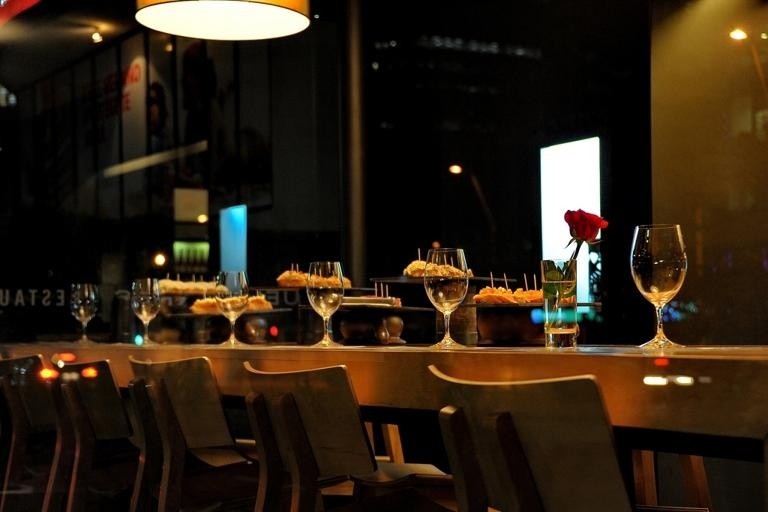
[543,207,609,321]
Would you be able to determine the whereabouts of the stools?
[0,354,49,510]
[127,354,254,507]
[50,355,125,509]
[241,359,452,507]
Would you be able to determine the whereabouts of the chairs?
[427,362,635,508]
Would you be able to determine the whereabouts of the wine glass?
[305,223,690,350]
[68,271,251,347]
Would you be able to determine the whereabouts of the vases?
[540,258,579,350]
